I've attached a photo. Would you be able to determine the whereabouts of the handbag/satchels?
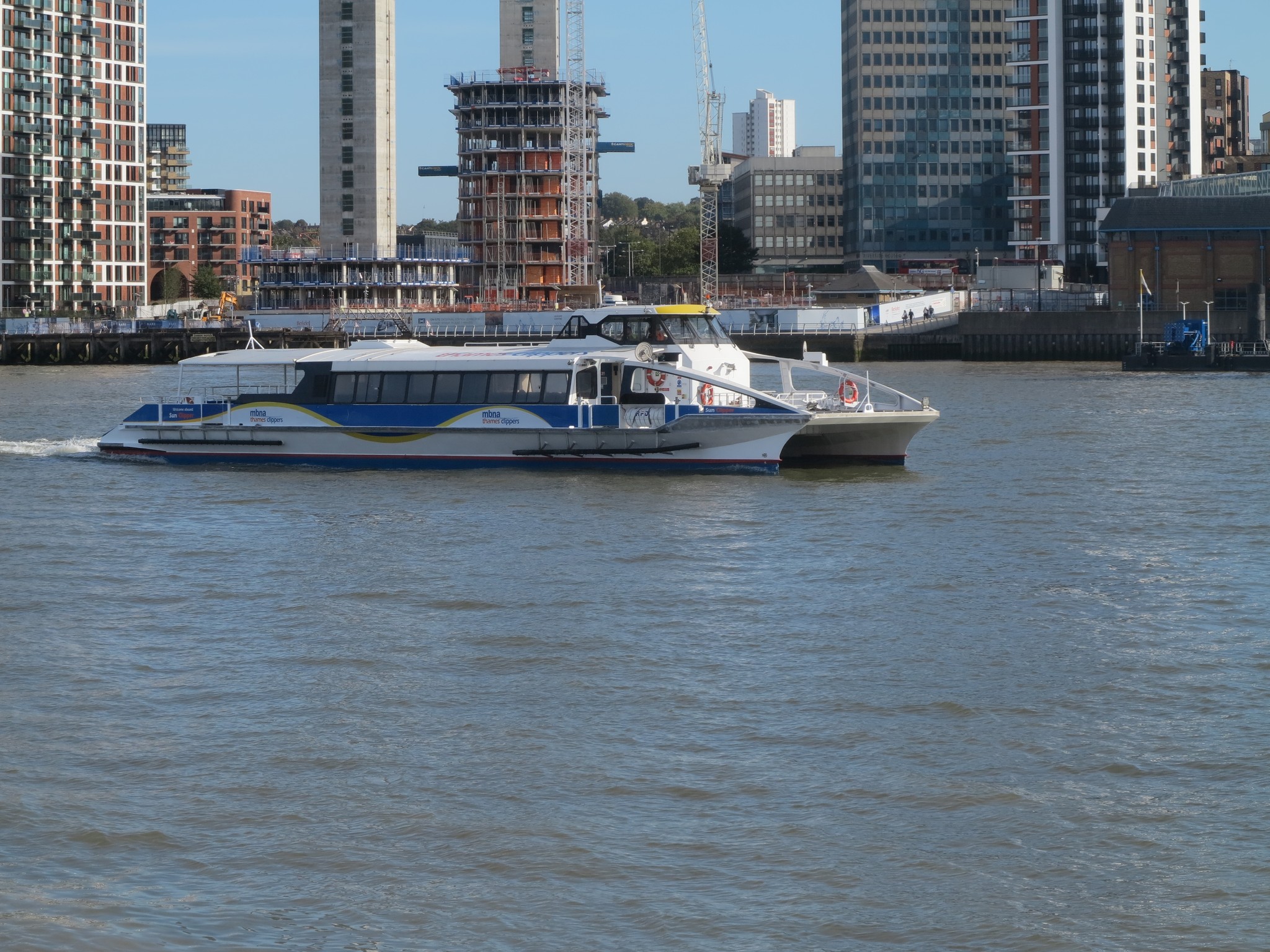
[902,317,904,319]
[933,313,935,318]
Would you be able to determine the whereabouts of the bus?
[898,256,970,275]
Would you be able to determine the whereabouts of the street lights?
[598,241,644,277]
[974,247,980,267]
[1203,300,1215,344]
[21,295,31,312]
[255,285,259,314]
[1179,301,1190,320]
[994,257,998,289]
[132,293,142,319]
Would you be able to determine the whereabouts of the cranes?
[690,0,732,311]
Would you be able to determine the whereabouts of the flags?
[1140,269,1152,296]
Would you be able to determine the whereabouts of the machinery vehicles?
[164,291,240,321]
[91,302,116,320]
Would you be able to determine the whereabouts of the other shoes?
[902,324,904,326]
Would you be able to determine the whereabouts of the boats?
[96,273,942,479]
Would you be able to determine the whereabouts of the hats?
[1025,306,1027,307]
[1001,305,1003,306]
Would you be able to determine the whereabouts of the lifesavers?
[839,380,857,404]
[700,383,713,405]
[646,369,665,386]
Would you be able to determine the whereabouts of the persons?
[901,310,908,326]
[655,324,668,341]
[999,304,1030,312]
[620,326,637,341]
[923,305,934,322]
[908,309,915,324]
